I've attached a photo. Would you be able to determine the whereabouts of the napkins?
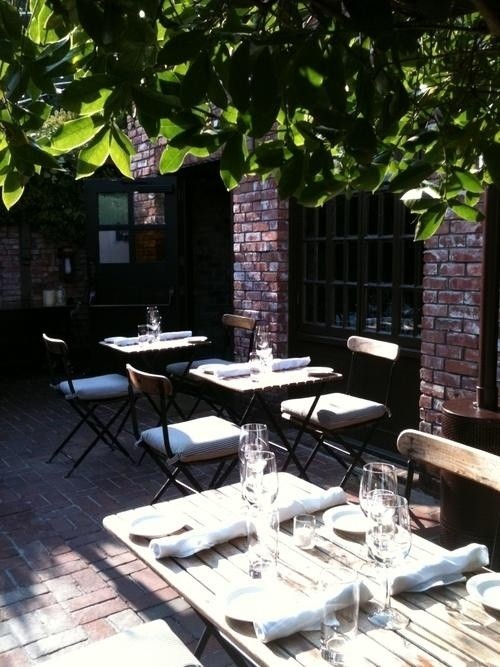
[266,484,345,525]
[252,581,374,645]
[158,330,192,340]
[114,336,140,347]
[146,515,257,563]
[216,361,255,380]
[388,536,489,602]
[271,355,312,372]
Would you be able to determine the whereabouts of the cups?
[320,569,363,666]
[137,307,161,348]
[248,325,273,383]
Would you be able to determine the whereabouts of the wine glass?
[238,423,412,629]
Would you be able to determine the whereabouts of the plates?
[184,335,208,343]
[127,512,191,538]
[466,569,500,613]
[304,366,335,376]
[218,584,304,623]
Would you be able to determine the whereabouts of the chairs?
[41,329,142,473]
[124,360,249,504]
[26,617,205,666]
[279,334,402,488]
[161,313,258,423]
[395,427,500,565]
